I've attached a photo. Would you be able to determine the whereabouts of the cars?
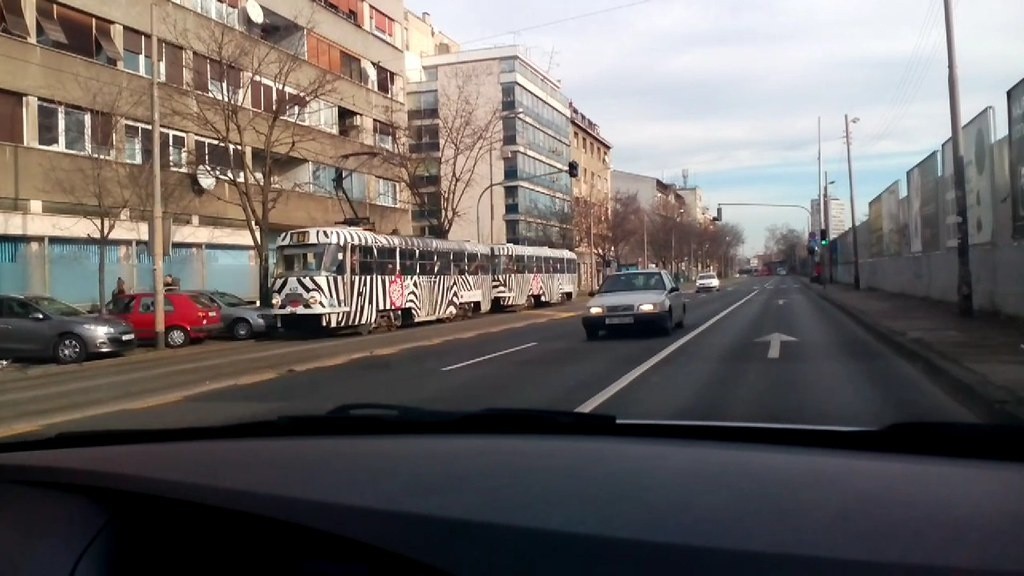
[581,269,686,342]
[167,290,276,341]
[696,272,721,293]
[85,286,224,347]
[0,293,137,365]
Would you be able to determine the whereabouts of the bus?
[270,227,578,337]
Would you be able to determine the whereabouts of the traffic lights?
[820,229,829,248]
[568,160,579,178]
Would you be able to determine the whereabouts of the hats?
[117,277,124,287]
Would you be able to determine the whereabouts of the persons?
[164,274,180,292]
[336,253,433,275]
[112,279,125,307]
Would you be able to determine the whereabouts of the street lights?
[844,116,861,291]
